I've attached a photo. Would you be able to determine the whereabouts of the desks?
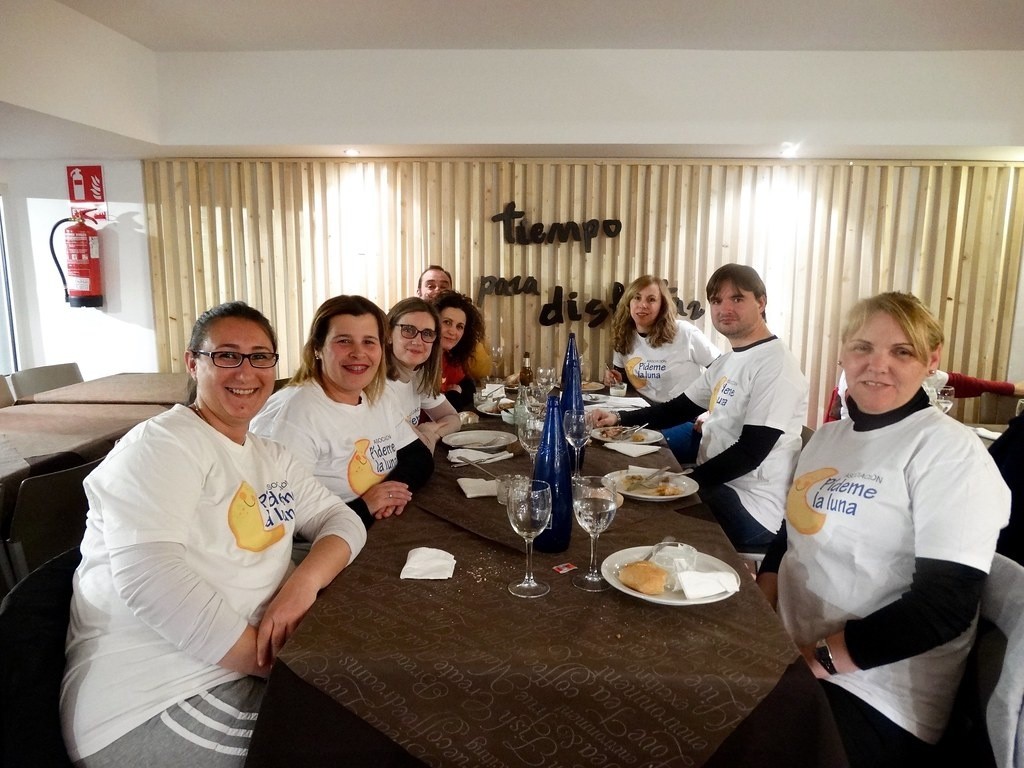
[0,373,192,590]
[963,424,1009,449]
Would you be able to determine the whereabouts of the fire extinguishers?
[50,208,102,308]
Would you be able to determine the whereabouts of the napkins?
[628,464,658,475]
[400,546,456,579]
[602,395,652,409]
[602,442,661,458]
[456,478,499,499]
[446,448,514,465]
[971,426,1002,441]
[482,383,506,399]
[673,571,740,600]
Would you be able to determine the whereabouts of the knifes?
[626,465,671,491]
[625,422,649,439]
[451,454,502,467]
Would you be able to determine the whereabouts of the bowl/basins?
[501,408,515,424]
[480,377,502,388]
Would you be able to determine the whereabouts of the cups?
[610,381,627,396]
[473,391,493,408]
[495,474,529,504]
[926,386,954,414]
[1016,399,1024,416]
[514,366,555,441]
[652,542,697,591]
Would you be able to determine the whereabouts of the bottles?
[519,352,533,404]
[560,333,585,471]
[532,396,572,553]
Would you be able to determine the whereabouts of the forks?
[452,438,498,447]
[638,468,694,489]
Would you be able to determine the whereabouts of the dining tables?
[244,378,850,768]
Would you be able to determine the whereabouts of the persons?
[585,264,809,545]
[417,265,492,381]
[384,297,461,457]
[755,292,1012,768]
[250,295,434,565]
[418,290,485,427]
[602,275,723,463]
[57,302,366,768]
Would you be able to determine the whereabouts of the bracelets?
[815,638,837,674]
[610,410,621,424]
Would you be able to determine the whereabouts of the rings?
[389,491,392,498]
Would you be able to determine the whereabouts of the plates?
[582,488,624,514]
[501,381,518,389]
[581,394,608,403]
[554,381,605,394]
[601,546,741,606]
[601,470,699,502]
[477,401,501,416]
[442,430,518,453]
[590,426,664,444]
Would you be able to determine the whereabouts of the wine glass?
[518,413,545,480]
[489,346,503,383]
[562,410,592,486]
[507,476,617,597]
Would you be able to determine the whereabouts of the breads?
[486,401,516,412]
[632,434,645,441]
[505,372,521,388]
[619,561,669,595]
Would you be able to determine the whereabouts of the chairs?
[0,363,109,768]
[737,424,815,560]
[932,552,1023,768]
[979,391,1024,425]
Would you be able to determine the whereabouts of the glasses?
[194,350,279,369]
[395,324,437,342]
